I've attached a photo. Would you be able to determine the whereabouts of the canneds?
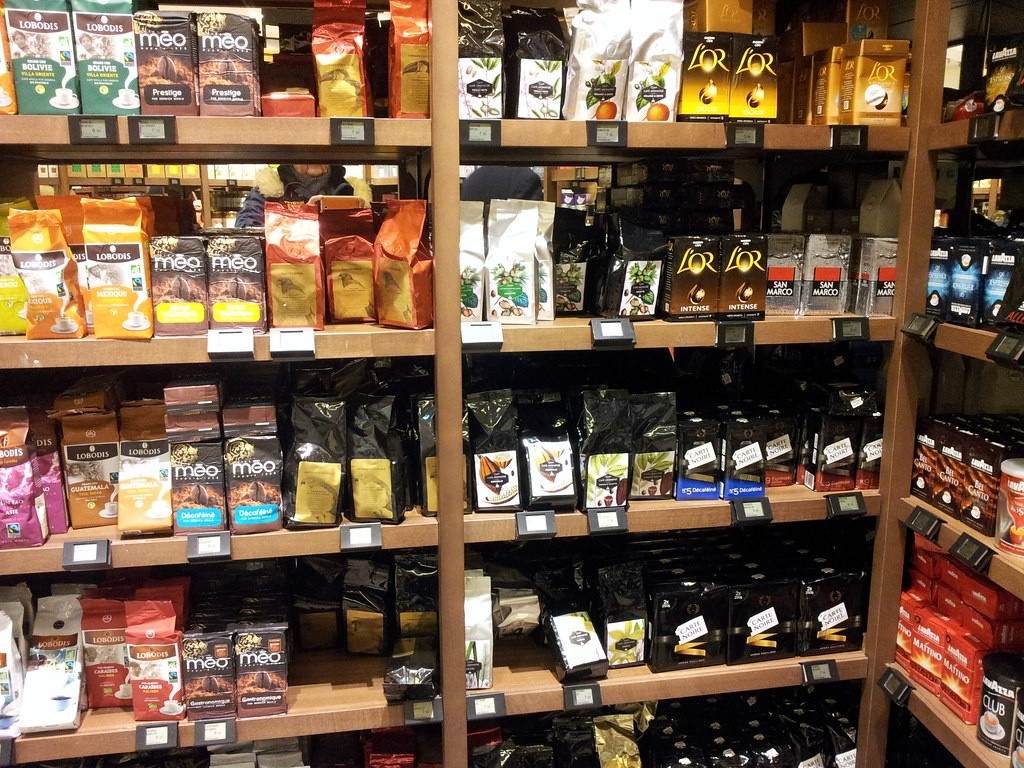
[976,651,1024,768]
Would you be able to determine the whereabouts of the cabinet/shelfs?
[452,1,918,768]
[852,0,1024,768]
[0,0,446,768]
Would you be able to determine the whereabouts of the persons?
[459,165,544,202]
[232,163,355,231]
